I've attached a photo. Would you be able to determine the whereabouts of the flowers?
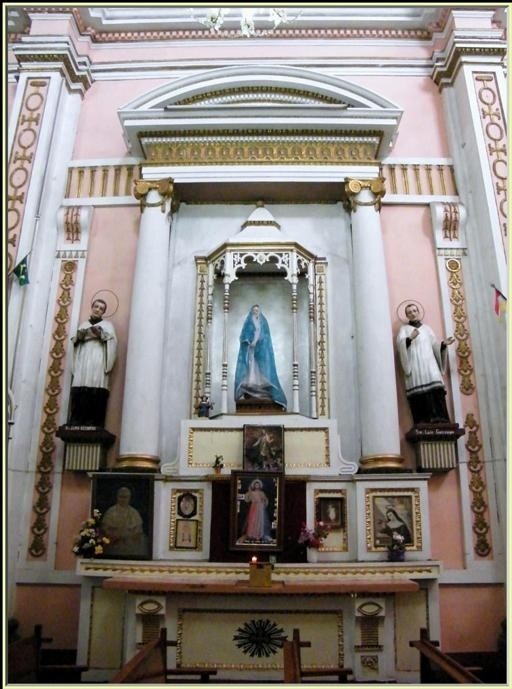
[72,516,120,558]
[297,521,332,549]
[387,532,407,551]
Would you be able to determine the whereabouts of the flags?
[14,256,30,287]
[493,288,506,324]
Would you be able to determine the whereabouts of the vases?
[389,551,405,562]
[306,547,318,563]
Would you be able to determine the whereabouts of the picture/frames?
[88,473,155,561]
[242,424,285,471]
[305,481,358,563]
[228,469,284,552]
[355,480,431,561]
[152,481,213,562]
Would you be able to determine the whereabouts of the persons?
[68,299,118,429]
[244,482,269,541]
[394,304,456,426]
[101,487,148,556]
[198,396,211,417]
[385,497,411,531]
[378,509,411,545]
[232,305,289,410]
[252,429,275,468]
